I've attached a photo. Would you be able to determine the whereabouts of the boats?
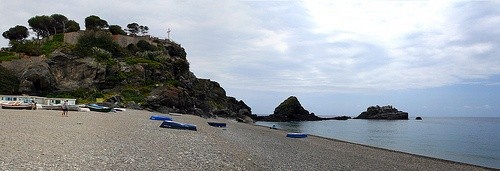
[159,120,196,130]
[207,122,227,127]
[0,95,80,110]
[88,106,113,112]
[286,133,309,138]
[150,115,173,120]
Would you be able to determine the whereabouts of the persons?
[61,101,66,115]
[64,100,69,117]
